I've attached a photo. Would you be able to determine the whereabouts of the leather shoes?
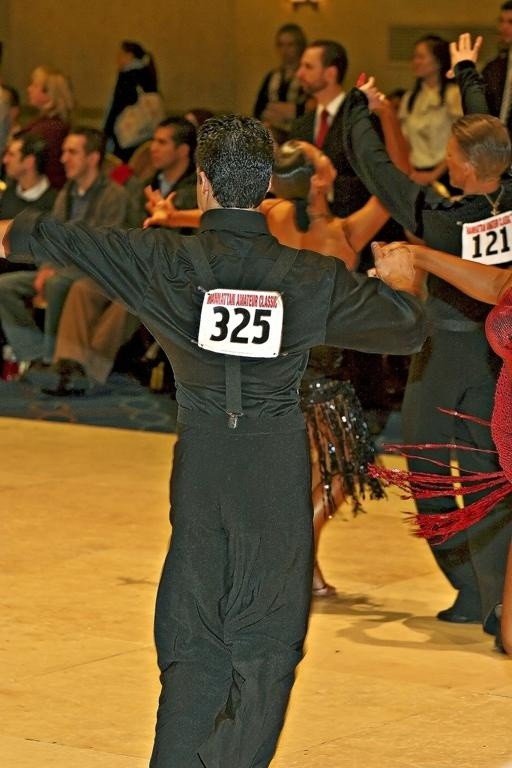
[438,608,480,623]
[483,602,505,652]
[21,359,86,397]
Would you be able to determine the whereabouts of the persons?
[0,0,511,387]
[0,111,430,767]
[144,31,512,658]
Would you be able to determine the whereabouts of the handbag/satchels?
[113,93,168,150]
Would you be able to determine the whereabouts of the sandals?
[313,563,335,596]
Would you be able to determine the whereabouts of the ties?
[315,110,328,149]
